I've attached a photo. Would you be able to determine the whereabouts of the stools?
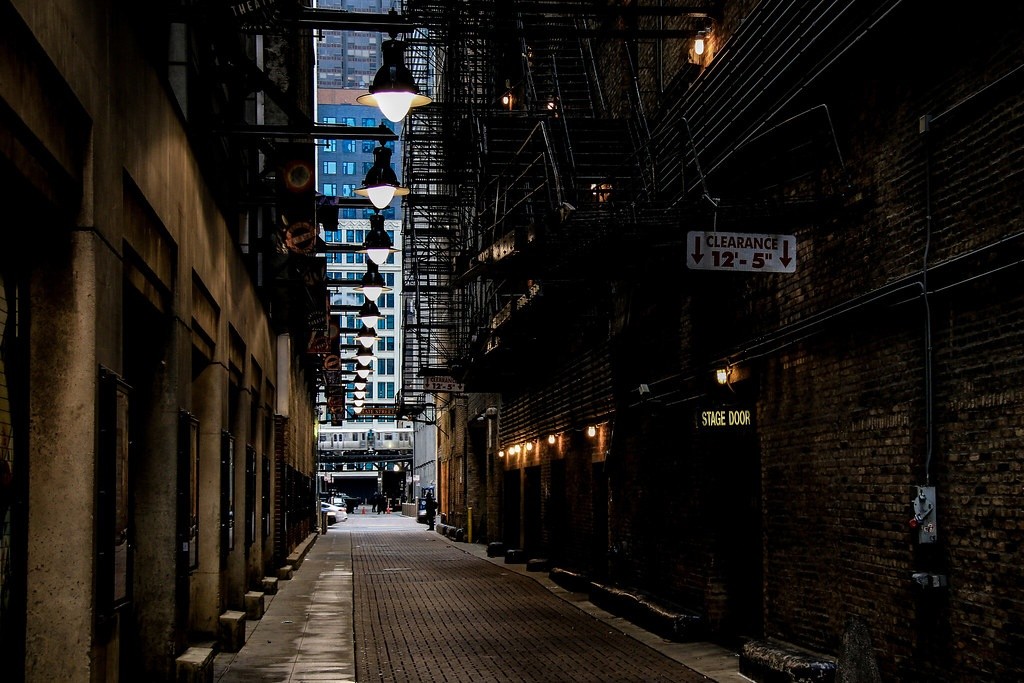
[175,564,293,683]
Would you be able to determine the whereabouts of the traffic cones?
[362,508,365,514]
[386,505,390,514]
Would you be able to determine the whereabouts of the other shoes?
[427,528,434,530]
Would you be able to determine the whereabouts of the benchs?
[286,532,318,571]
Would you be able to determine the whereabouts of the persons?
[420,493,436,530]
[372,492,407,515]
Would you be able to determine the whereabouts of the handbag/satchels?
[433,502,438,509]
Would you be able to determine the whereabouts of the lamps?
[208,0,435,415]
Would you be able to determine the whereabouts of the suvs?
[329,493,358,513]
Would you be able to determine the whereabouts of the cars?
[319,502,350,526]
[328,497,347,508]
